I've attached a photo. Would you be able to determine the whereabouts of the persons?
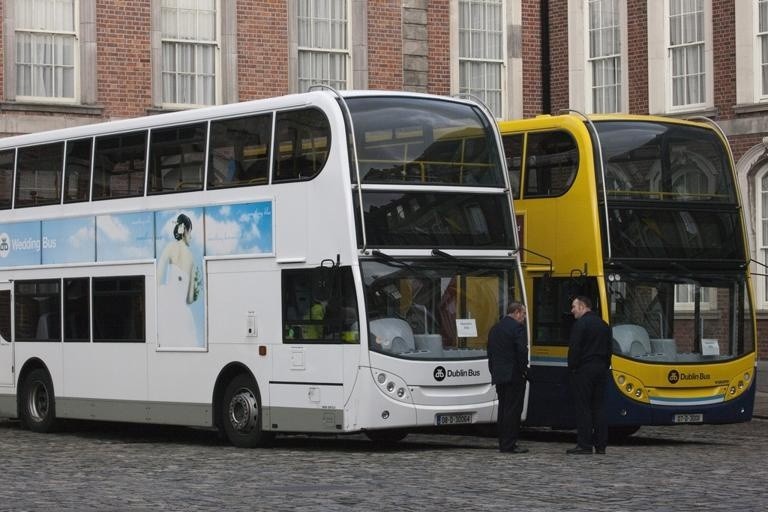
[487,302,530,452]
[157,212,199,348]
[565,294,613,454]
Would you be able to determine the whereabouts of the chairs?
[622,285,671,340]
[137,154,421,190]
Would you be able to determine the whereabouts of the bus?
[242,108,757,437]
[0,85,533,447]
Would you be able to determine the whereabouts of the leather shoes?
[597,448,605,454]
[510,447,530,453]
[567,445,593,455]
